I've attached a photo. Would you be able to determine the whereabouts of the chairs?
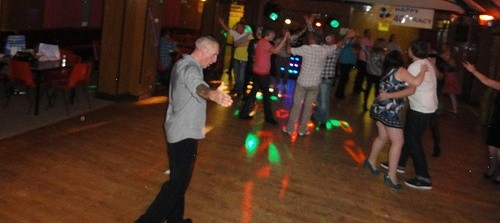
[0,47,92,116]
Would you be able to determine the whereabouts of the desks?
[29,61,72,116]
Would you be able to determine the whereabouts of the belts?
[322,77,334,80]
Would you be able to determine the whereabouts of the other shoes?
[265,118,278,125]
[298,132,310,136]
[238,115,254,120]
[310,116,327,128]
[432,145,441,157]
[281,129,290,134]
[167,217,192,223]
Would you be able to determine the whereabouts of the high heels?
[483,169,496,178]
[384,174,401,190]
[364,160,381,174]
[492,176,500,184]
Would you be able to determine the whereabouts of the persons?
[133,35,233,223]
[156,12,468,157]
[462,60,500,184]
[375,40,438,190]
[364,51,429,189]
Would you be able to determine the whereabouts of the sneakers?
[381,161,405,173]
[404,177,432,189]
